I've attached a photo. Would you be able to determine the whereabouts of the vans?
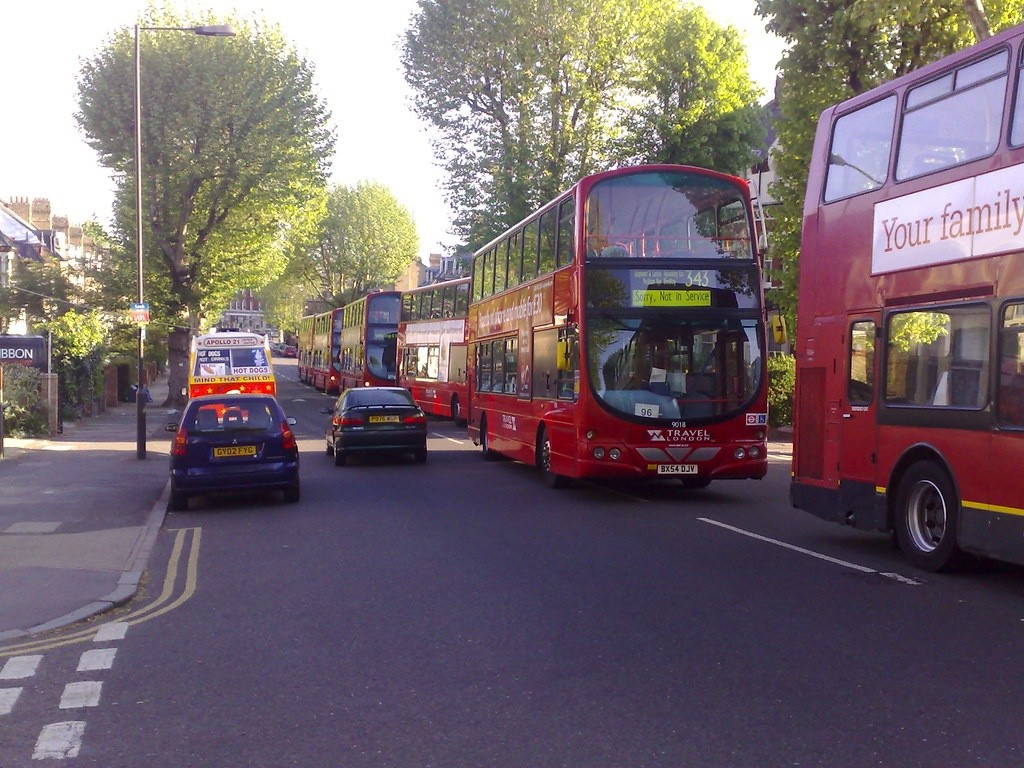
[186,329,278,424]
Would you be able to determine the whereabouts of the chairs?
[247,410,269,426]
[523,241,724,281]
[480,371,575,400]
[197,409,218,426]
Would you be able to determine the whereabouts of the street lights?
[131,23,238,460]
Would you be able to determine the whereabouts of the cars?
[163,392,301,510]
[320,386,429,465]
[270,341,296,359]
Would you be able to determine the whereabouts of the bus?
[789,18,1024,572]
[309,307,344,395]
[297,312,319,385]
[464,162,787,492]
[339,291,402,397]
[396,276,473,426]
